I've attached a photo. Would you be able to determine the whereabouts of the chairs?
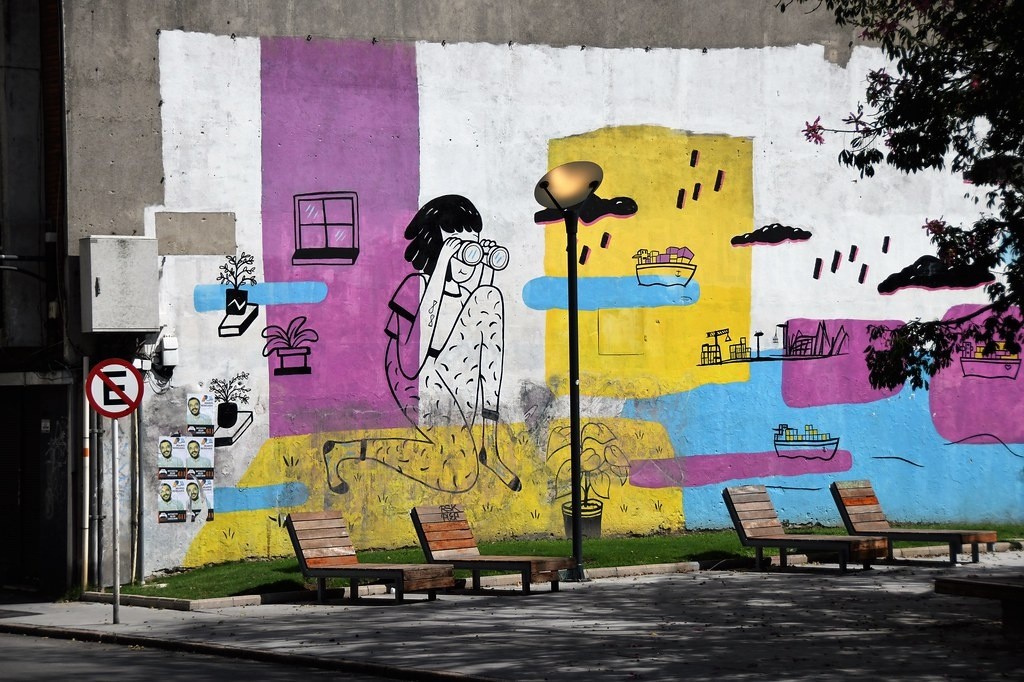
[410,504,575,595]
[829,479,997,563]
[721,485,888,570]
[283,509,456,604]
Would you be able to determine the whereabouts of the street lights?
[534,160,604,582]
